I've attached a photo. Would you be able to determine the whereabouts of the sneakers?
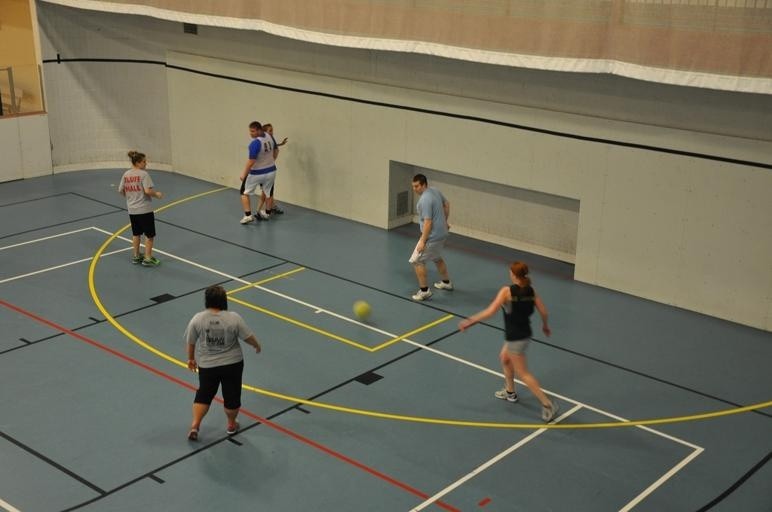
[239,213,255,224]
[131,256,144,264]
[253,211,263,219]
[141,256,161,267]
[271,204,284,214]
[226,418,240,435]
[434,280,453,290]
[541,401,560,423]
[187,425,200,440]
[259,210,270,220]
[411,287,433,301]
[494,390,518,403]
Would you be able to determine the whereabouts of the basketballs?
[352,299,372,321]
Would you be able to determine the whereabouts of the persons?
[408,173,454,302]
[183,286,261,440]
[261,124,288,216]
[118,151,164,267]
[240,121,276,226]
[459,263,559,421]
